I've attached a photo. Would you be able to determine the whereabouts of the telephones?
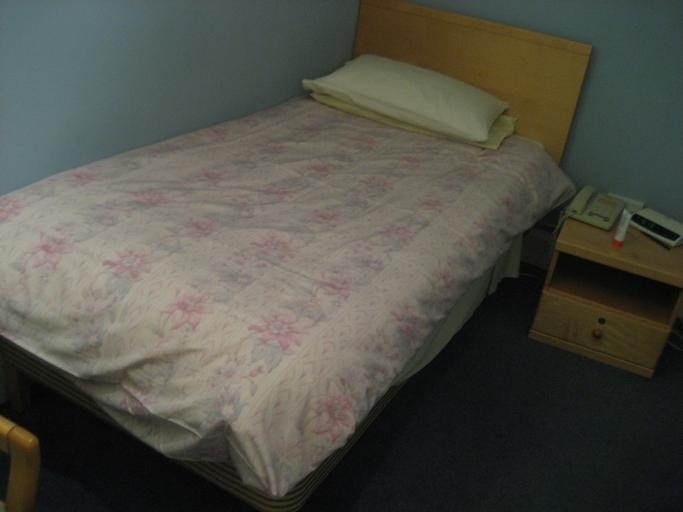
[553,184,622,236]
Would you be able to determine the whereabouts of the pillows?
[303,53,509,142]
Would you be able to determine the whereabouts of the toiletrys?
[613,210,631,250]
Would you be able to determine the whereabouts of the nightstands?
[529,215,683,379]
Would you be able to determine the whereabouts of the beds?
[0,2,594,511]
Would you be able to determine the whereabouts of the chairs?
[0,417,43,512]
